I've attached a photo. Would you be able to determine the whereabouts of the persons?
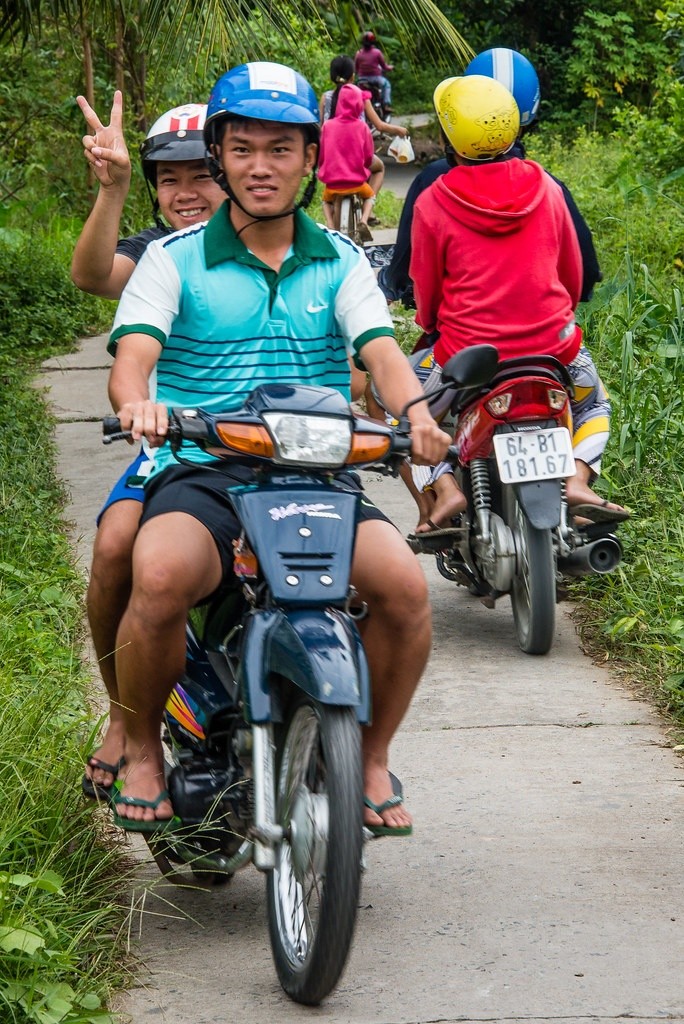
[318,84,373,243]
[316,55,410,227]
[354,31,396,114]
[377,48,632,541]
[68,60,454,838]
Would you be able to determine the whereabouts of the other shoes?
[383,106,397,114]
[367,215,382,227]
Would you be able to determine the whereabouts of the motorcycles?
[102,344,501,1008]
[363,244,625,657]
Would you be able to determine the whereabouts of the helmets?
[431,74,522,164]
[464,45,542,132]
[140,103,216,180]
[361,30,377,45]
[201,60,323,160]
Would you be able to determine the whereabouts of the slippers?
[356,222,374,243]
[405,520,472,541]
[357,783,414,840]
[80,740,126,798]
[571,497,631,525]
[110,778,180,833]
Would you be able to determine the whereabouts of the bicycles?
[333,197,376,236]
